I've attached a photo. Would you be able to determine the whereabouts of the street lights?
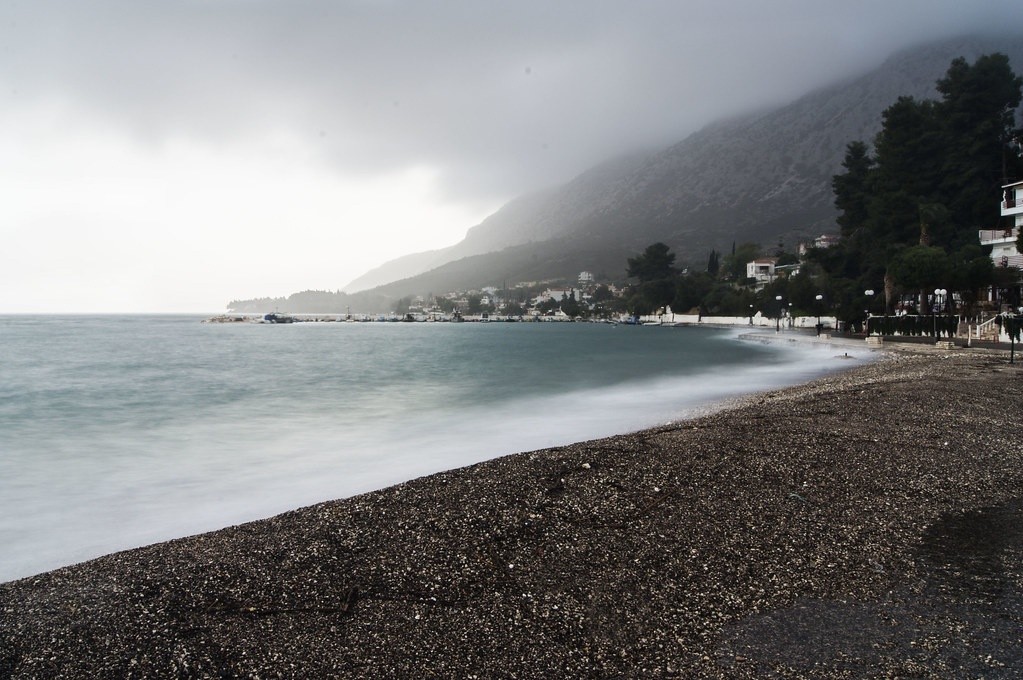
[864,289,875,338]
[749,304,754,326]
[788,302,793,328]
[815,295,823,337]
[775,295,782,334]
[934,288,947,342]
